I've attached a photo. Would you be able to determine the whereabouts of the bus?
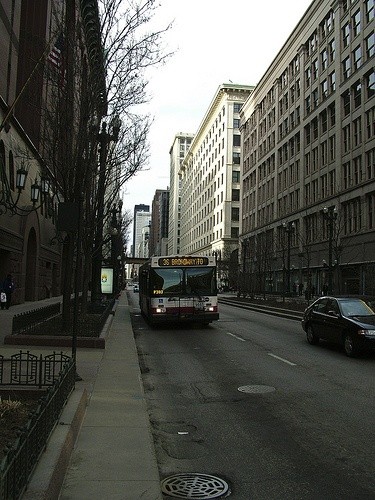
[138,254,219,327]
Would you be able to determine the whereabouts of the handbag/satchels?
[1,293,7,302]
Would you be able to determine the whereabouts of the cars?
[300,292,375,358]
[128,281,140,293]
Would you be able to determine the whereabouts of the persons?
[1,274,13,310]
[298,281,304,296]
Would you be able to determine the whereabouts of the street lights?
[241,236,252,289]
[88,108,119,308]
[281,222,297,294]
[322,203,339,295]
